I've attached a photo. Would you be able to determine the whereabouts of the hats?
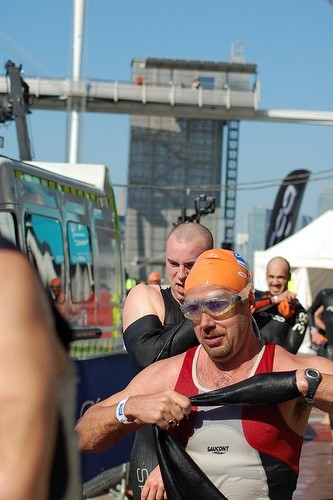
[148,271,161,282]
[184,249,254,301]
[91,283,111,292]
[51,278,62,286]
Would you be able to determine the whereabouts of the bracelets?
[274,295,278,304]
[116,396,133,424]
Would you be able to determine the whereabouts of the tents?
[252,208,333,356]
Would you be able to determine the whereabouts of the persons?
[252,256,313,441]
[307,288,333,441]
[0,231,68,500]
[123,223,214,500]
[49,270,163,336]
[73,249,333,500]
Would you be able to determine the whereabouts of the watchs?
[304,368,323,404]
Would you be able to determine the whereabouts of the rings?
[168,418,174,425]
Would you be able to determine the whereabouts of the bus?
[0,153,129,361]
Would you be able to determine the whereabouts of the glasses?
[180,282,253,320]
[50,285,60,289]
